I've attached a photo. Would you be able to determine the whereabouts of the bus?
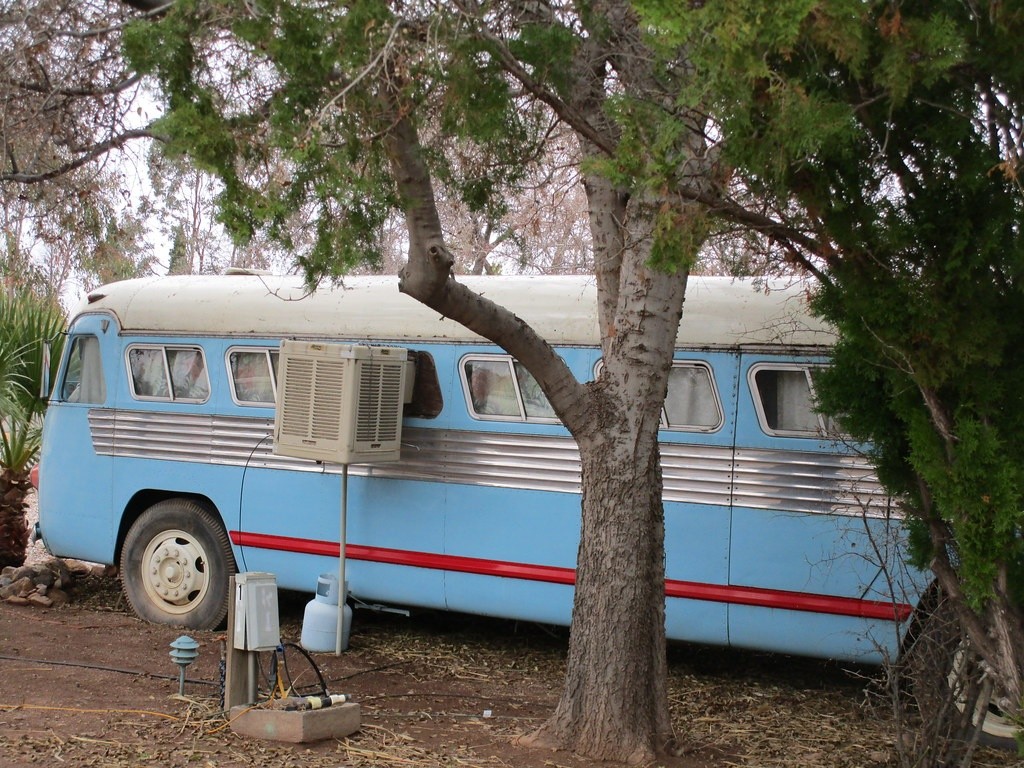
[39,265,1022,756]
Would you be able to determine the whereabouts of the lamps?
[167,636,200,667]
